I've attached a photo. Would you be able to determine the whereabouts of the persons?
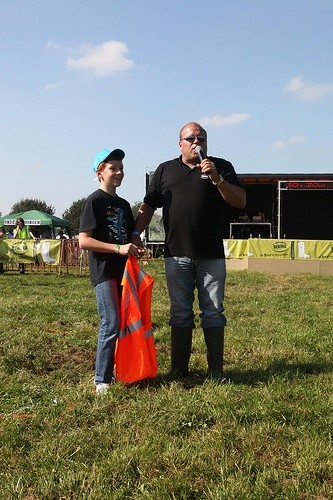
[132,122,248,382]
[78,149,145,399]
[0,218,69,273]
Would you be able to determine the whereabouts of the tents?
[0,210,71,239]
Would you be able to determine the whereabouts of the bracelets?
[132,231,141,238]
[115,244,119,254]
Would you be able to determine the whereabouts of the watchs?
[212,174,224,186]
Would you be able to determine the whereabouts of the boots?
[203,327,224,384]
[164,326,192,384]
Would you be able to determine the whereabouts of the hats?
[91,149,125,174]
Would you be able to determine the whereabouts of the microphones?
[195,146,211,175]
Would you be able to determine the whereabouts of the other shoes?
[94,382,112,397]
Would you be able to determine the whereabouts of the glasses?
[180,136,207,143]
